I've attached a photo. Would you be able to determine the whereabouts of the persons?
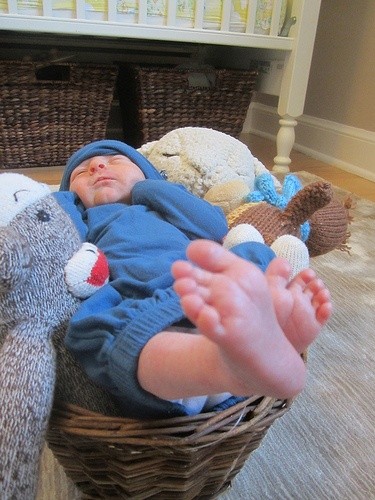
[58,140,333,421]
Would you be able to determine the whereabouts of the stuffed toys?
[136,126,355,282]
[0,173,110,500]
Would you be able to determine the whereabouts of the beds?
[0,1,318,174]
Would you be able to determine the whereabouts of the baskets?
[45,347,308,500]
[136,67,257,147]
[0,61,118,169]
